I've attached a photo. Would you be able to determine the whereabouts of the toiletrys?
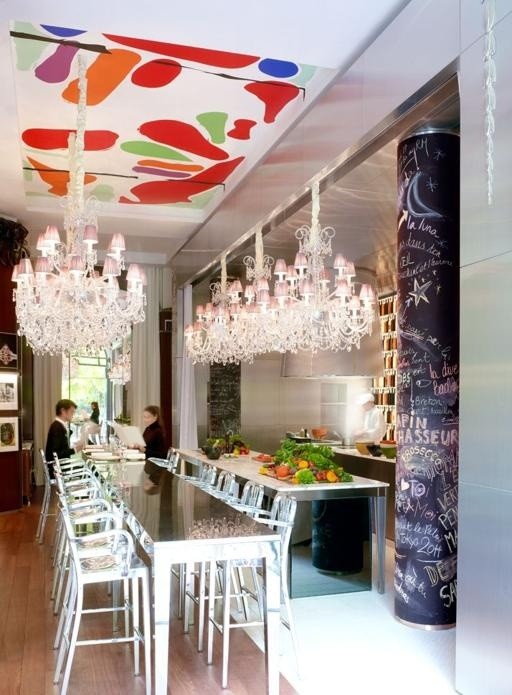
[300,425,305,437]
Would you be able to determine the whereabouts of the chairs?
[88,420,103,445]
[34,447,297,694]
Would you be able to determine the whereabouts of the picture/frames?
[0,416,21,455]
[0,372,21,416]
[0,331,20,371]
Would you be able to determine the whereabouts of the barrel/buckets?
[311,499,364,574]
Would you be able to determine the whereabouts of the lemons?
[298,460,308,468]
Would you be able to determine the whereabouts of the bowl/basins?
[367,444,382,457]
[355,442,375,456]
[380,444,397,459]
[205,447,222,460]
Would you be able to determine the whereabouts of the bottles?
[300,426,307,437]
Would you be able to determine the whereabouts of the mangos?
[327,471,338,482]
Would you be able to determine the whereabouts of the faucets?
[304,429,308,437]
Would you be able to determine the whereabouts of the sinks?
[289,438,335,444]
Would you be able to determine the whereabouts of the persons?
[127,405,167,460]
[142,460,165,496]
[45,398,85,480]
[90,401,101,423]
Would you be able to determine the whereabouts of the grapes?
[295,468,315,484]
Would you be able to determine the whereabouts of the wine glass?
[108,433,128,467]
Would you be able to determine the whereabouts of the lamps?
[184,180,380,366]
[9,51,150,359]
